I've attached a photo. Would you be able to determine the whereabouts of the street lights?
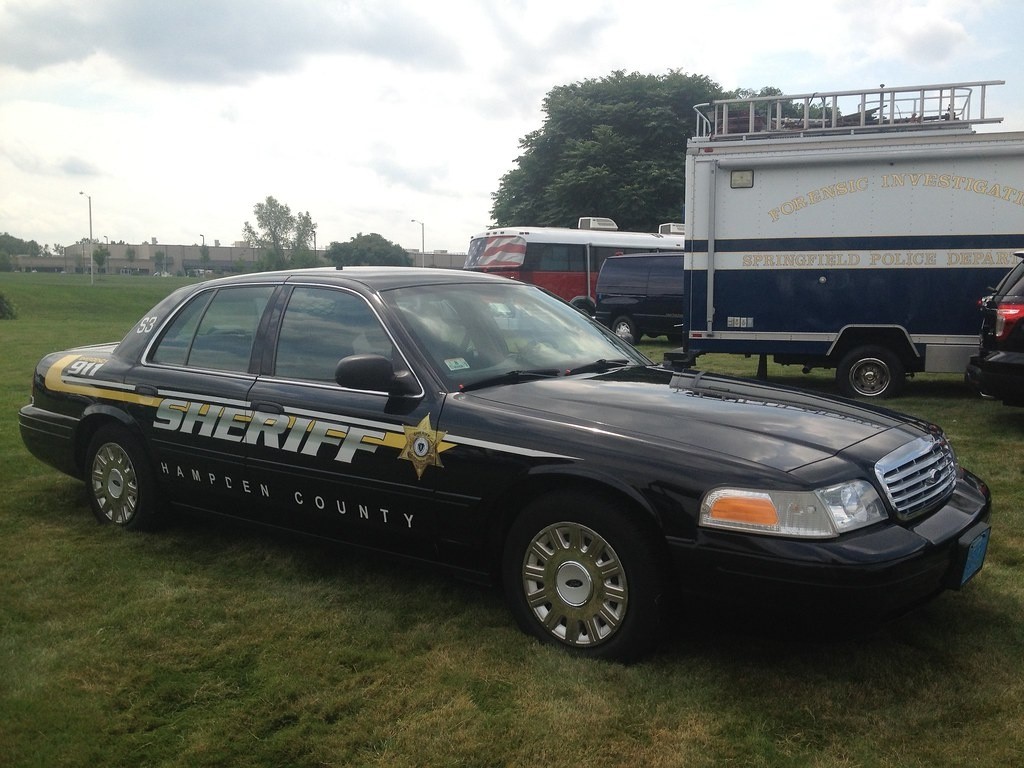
[79,189,94,282]
[410,219,426,269]
[200,234,205,268]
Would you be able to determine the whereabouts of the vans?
[594,251,687,348]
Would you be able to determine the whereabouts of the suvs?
[963,250,1024,407]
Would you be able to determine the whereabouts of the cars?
[17,266,994,661]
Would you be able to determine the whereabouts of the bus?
[463,217,685,334]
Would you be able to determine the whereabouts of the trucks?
[661,80,1024,402]
